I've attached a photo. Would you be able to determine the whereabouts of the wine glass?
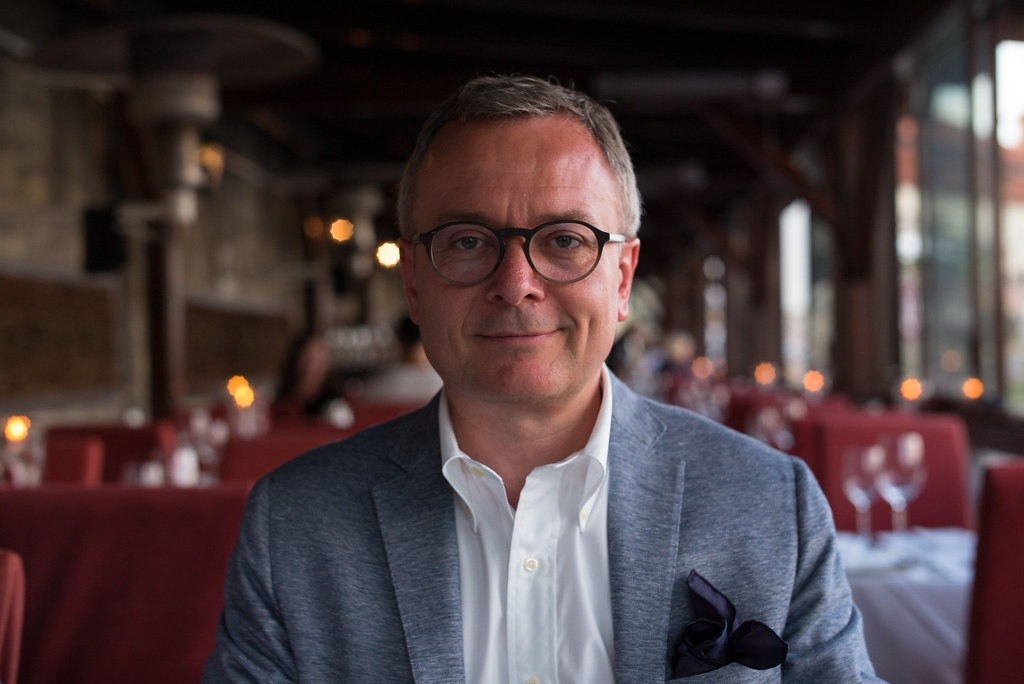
[878,433,929,549]
[843,446,885,549]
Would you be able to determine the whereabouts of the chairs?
[0,376,1023,684]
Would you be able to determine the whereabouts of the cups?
[9,416,43,491]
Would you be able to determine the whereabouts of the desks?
[835,525,975,684]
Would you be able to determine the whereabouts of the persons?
[276,275,759,426]
[199,74,893,684]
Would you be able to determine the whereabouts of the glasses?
[411,219,628,286]
